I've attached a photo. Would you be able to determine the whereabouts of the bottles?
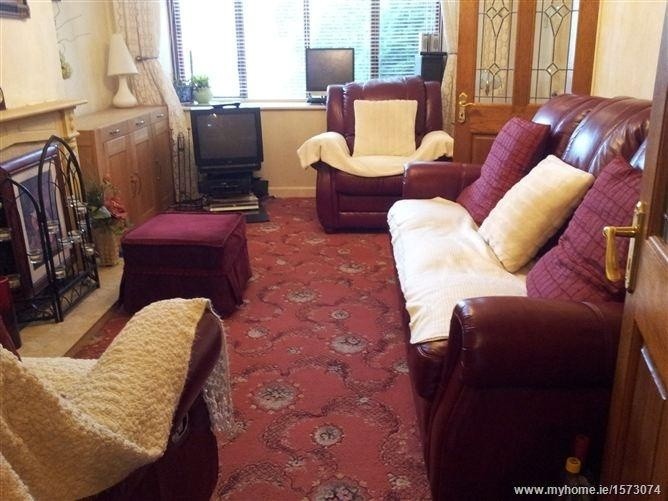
[552,456,590,501]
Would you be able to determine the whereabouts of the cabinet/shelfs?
[75,105,160,247]
[151,106,175,215]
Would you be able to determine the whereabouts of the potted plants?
[191,75,212,104]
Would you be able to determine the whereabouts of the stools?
[122,211,253,315]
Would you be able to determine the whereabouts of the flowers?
[79,174,127,226]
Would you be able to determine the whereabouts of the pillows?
[352,100,418,156]
[456,116,551,227]
[478,154,595,274]
[526,153,642,301]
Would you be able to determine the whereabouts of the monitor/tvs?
[190,107,264,170]
[306,48,354,94]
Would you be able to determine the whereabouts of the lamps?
[107,33,138,108]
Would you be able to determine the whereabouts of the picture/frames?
[0,144,77,302]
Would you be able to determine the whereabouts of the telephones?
[306,92,327,103]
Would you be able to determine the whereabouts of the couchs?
[387,94,654,501]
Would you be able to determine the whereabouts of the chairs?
[296,76,453,234]
[0,299,222,501]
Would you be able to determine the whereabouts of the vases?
[92,224,119,266]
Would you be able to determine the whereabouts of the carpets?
[61,198,433,500]
